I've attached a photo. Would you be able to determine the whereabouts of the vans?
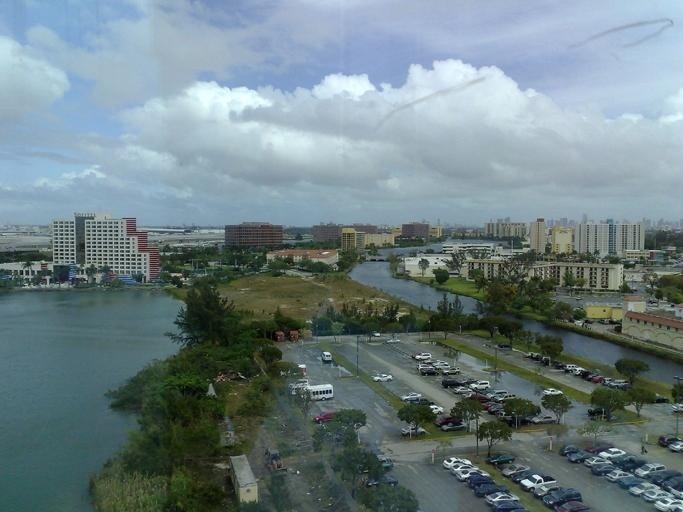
[321,352,332,361]
[288,378,308,388]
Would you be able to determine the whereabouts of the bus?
[303,384,334,401]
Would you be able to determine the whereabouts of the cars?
[371,373,392,383]
[672,403,682,411]
[560,435,682,512]
[370,330,380,337]
[497,342,510,348]
[564,363,669,405]
[363,476,398,487]
[399,353,564,436]
[443,452,581,510]
[572,318,620,330]
[549,295,560,302]
[575,296,582,300]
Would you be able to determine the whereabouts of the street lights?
[428,319,431,339]
[673,375,682,439]
[493,344,497,370]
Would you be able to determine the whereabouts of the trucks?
[312,411,337,423]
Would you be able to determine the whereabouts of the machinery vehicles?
[263,447,287,476]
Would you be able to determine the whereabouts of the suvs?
[383,337,400,344]
[361,456,392,472]
